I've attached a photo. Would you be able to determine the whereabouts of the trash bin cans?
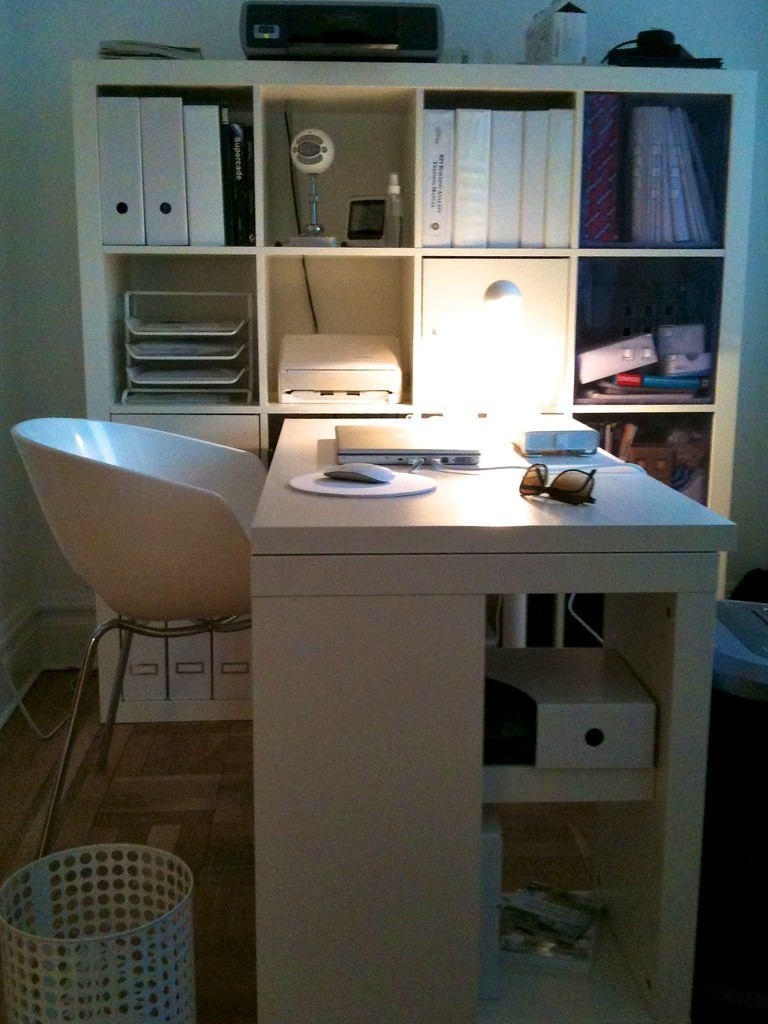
[2,842,199,1024]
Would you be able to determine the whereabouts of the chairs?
[9,417,267,862]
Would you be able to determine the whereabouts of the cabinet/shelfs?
[72,59,758,723]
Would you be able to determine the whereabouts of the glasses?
[519,463,597,507]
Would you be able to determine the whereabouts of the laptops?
[335,425,481,465]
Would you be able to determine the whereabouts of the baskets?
[0,843,198,1024]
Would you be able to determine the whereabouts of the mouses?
[323,464,396,484]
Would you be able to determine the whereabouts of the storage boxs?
[525,0,588,65]
[657,352,716,378]
[494,646,658,770]
[488,111,520,248]
[452,108,487,248]
[97,97,145,246]
[519,110,544,248]
[139,98,189,246]
[479,909,501,1000]
[421,109,451,247]
[576,333,659,384]
[543,109,569,248]
[655,322,706,355]
[182,105,225,246]
[480,804,502,909]
[221,124,256,247]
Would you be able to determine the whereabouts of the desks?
[249,419,735,1024]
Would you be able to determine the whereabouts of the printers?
[278,333,403,405]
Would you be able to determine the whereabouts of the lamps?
[484,281,522,316]
[289,129,336,247]
